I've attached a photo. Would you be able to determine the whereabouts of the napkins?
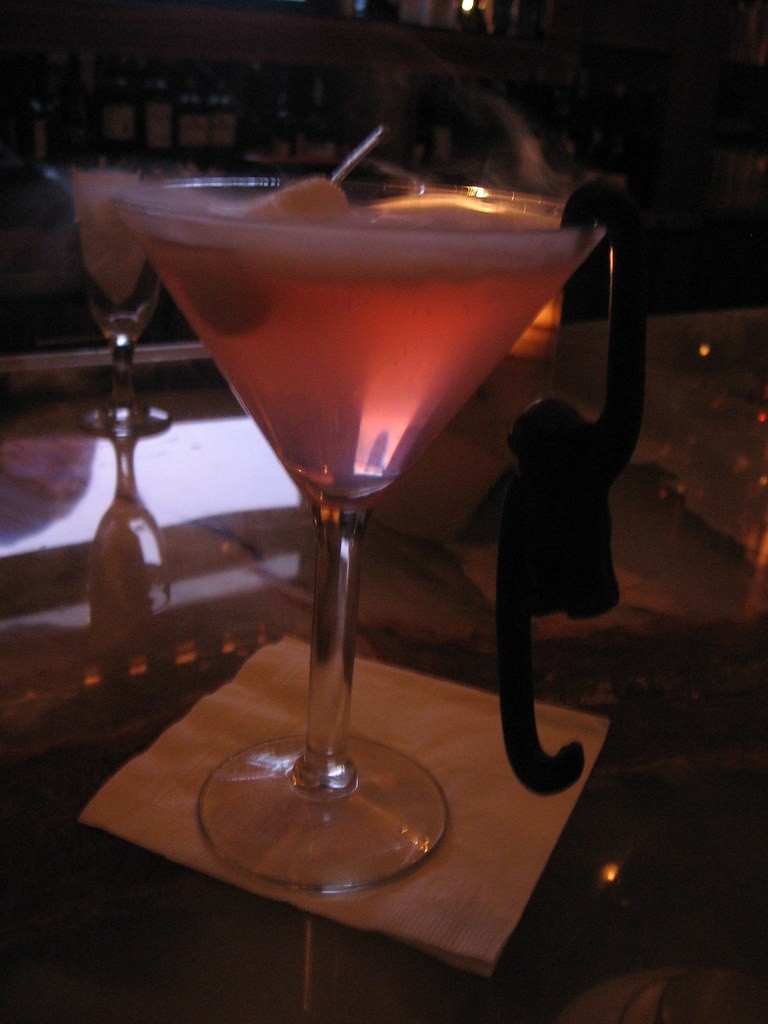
[77,635,611,977]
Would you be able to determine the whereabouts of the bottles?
[14,51,350,175]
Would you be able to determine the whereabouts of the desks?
[1,306,767,1024]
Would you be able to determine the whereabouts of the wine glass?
[116,175,605,893]
[71,167,171,440]
